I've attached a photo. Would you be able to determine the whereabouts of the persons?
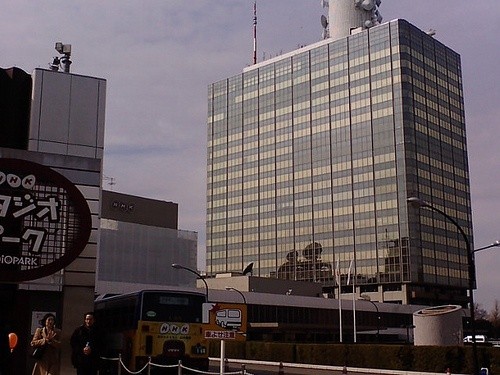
[30,313,64,374]
[70,312,101,375]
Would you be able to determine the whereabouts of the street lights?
[225,286,247,305]
[406,196,500,375]
[171,263,208,304]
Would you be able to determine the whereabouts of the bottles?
[85,342,89,354]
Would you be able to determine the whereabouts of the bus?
[96,290,209,375]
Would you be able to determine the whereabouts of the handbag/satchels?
[31,326,48,361]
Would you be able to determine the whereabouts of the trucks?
[463,335,484,343]
